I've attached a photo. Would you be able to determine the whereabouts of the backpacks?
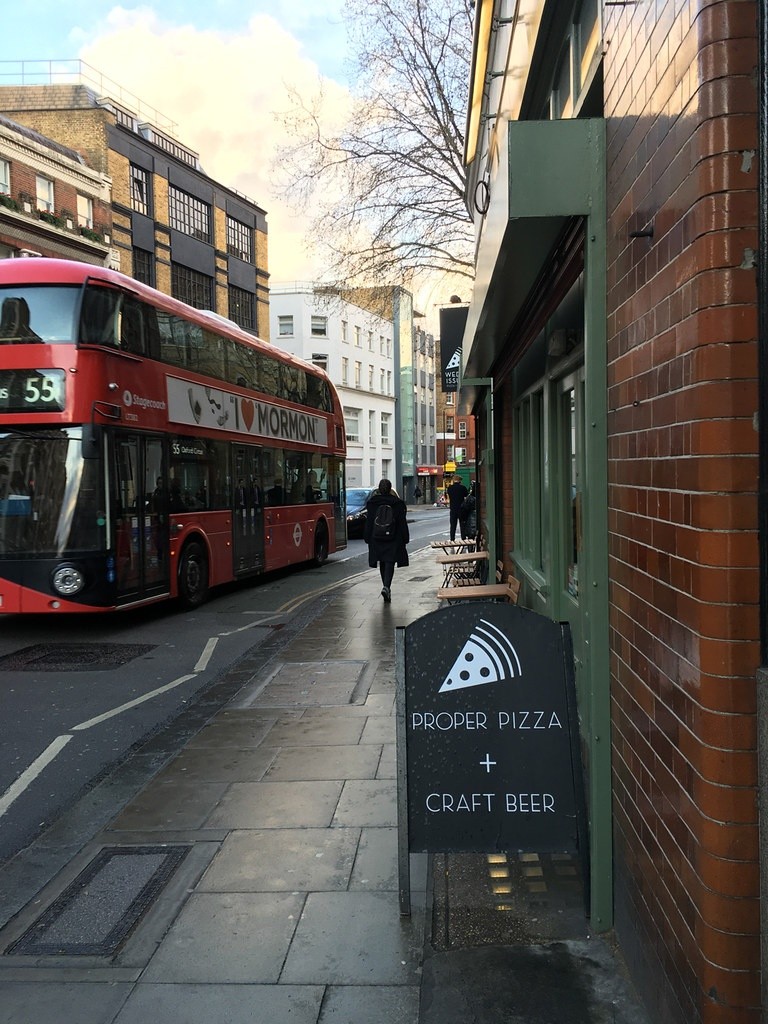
[372,497,398,541]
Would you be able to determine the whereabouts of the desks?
[435,582,510,610]
[431,539,477,554]
[436,550,487,587]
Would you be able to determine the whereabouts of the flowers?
[40,205,66,227]
[0,192,21,211]
[75,223,104,242]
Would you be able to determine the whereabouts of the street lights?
[442,408,462,507]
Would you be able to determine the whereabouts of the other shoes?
[381,587,391,603]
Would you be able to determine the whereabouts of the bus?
[0,256,349,614]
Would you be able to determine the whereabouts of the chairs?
[452,561,489,588]
[496,559,503,583]
[505,575,521,606]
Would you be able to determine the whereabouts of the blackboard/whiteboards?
[396,602,590,853]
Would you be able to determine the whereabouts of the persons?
[235,477,263,530]
[364,479,410,603]
[289,468,321,504]
[413,486,421,501]
[447,475,469,541]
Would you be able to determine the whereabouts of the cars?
[341,486,401,538]
